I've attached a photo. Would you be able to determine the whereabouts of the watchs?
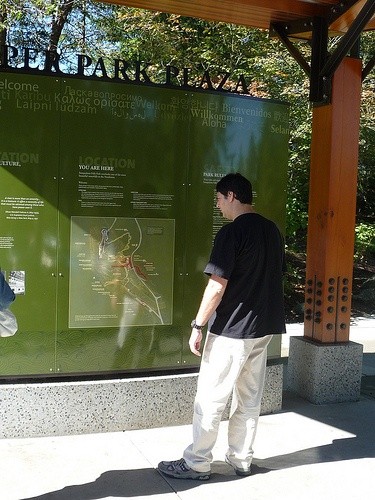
[190,320,205,329]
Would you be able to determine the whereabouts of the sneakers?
[158,458,211,480]
[225,455,251,476]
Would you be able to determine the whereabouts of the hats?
[215,173,253,205]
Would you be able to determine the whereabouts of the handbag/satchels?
[0,308,18,338]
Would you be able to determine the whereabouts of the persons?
[157,173,287,480]
